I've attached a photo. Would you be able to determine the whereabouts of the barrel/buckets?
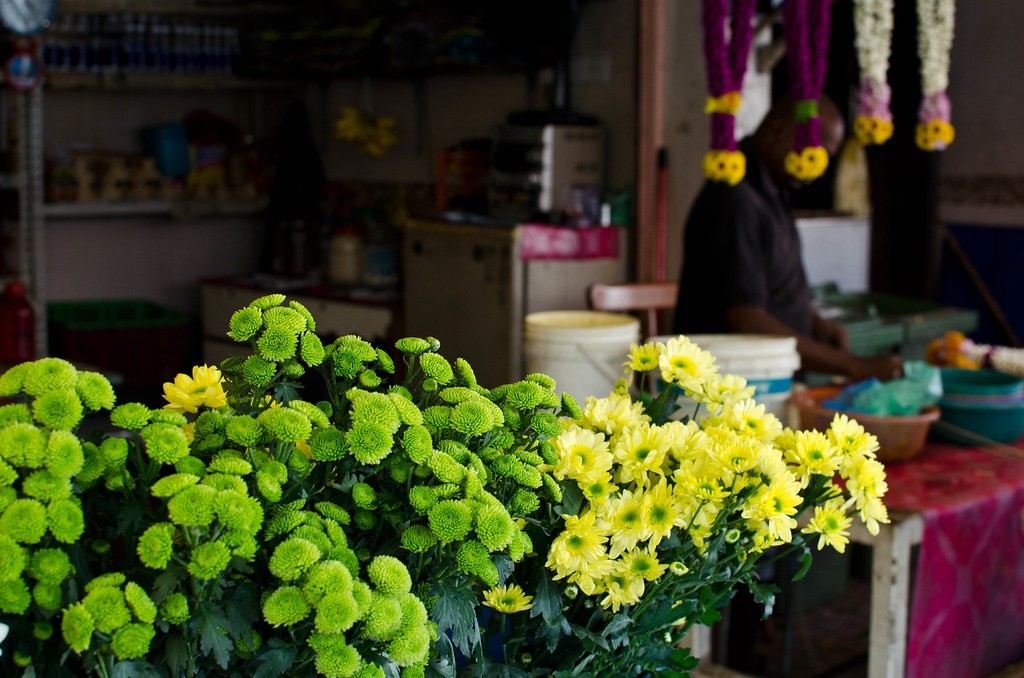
[654,331,802,426]
[523,309,642,405]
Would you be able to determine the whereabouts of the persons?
[671,81,904,383]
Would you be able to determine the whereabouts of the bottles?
[1,279,38,377]
[328,227,362,282]
[32,9,275,77]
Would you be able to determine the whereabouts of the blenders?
[484,53,607,221]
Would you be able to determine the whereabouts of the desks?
[780,436,1022,678]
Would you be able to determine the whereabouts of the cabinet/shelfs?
[17,59,288,367]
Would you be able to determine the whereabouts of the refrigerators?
[396,216,629,390]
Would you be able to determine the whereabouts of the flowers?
[702,2,957,185]
[0,294,890,677]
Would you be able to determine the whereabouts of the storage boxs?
[523,309,641,400]
[935,369,1024,443]
[646,332,800,431]
[787,388,943,460]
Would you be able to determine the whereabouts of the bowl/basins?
[786,280,1024,460]
